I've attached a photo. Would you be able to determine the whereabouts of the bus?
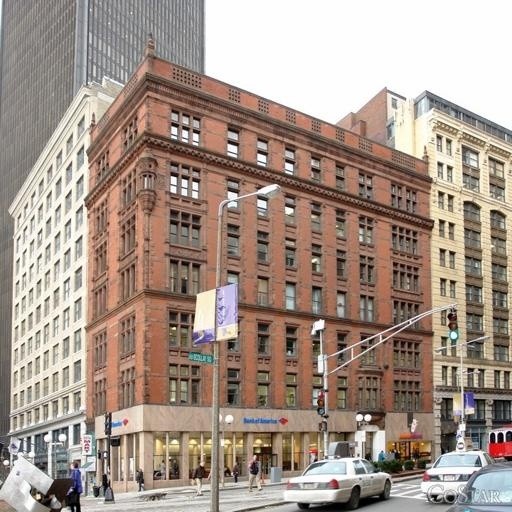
[484,420,512,460]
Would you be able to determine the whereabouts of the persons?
[193,462,206,496]
[232,463,239,482]
[161,460,166,480]
[378,449,401,461]
[136,469,143,492]
[173,459,177,479]
[248,455,263,492]
[69,462,83,512]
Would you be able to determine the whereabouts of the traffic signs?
[187,351,214,364]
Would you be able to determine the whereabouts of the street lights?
[219,398,234,487]
[3,457,18,473]
[356,412,373,457]
[17,438,46,453]
[207,182,284,512]
[18,451,36,463]
[42,432,68,479]
[435,335,492,434]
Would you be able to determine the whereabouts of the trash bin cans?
[94,487,99,497]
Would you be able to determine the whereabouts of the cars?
[420,447,497,504]
[439,460,512,512]
[279,456,394,510]
[154,469,163,480]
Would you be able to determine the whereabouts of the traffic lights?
[103,410,110,436]
[447,309,459,341]
[102,450,109,458]
[318,421,327,432]
[97,450,102,459]
[315,389,326,417]
